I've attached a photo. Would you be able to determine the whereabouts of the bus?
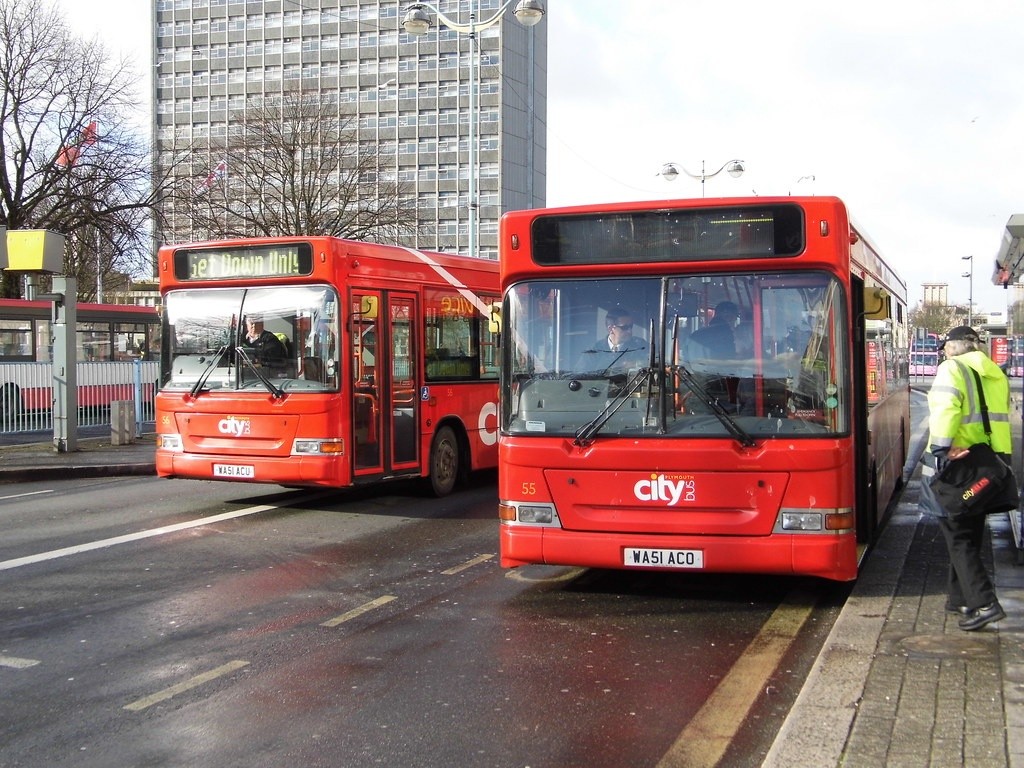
[1,296,164,424]
[487,196,911,583]
[155,237,558,500]
[910,333,942,376]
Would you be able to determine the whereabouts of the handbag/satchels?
[928,441,1018,533]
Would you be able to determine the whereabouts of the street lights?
[400,0,546,259]
[962,255,972,328]
[655,157,746,198]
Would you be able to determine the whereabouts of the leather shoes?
[957,602,1005,632]
[945,598,964,614]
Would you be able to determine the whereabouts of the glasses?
[614,323,634,330]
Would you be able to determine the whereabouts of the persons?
[919,326,1015,631]
[573,307,650,378]
[218,309,288,378]
[677,300,789,418]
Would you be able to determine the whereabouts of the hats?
[933,326,986,348]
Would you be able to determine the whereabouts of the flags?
[191,159,225,200]
[56,119,96,165]
